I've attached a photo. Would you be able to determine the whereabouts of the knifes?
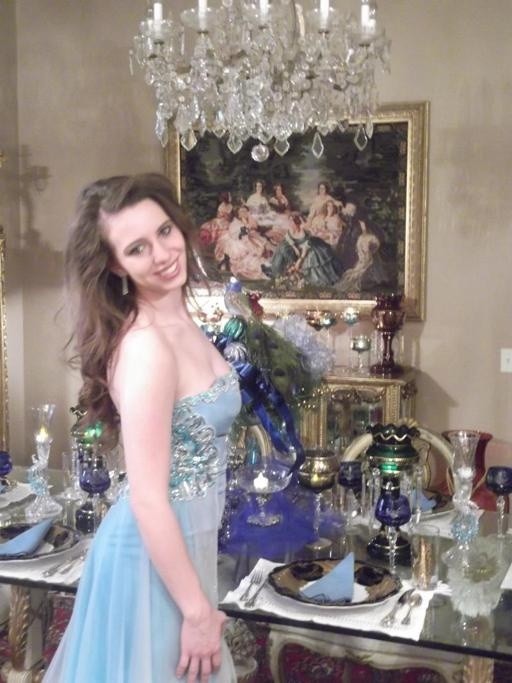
[379,588,416,626]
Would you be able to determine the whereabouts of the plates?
[0,520,82,565]
[270,558,402,609]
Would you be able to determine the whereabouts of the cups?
[410,525,440,589]
[62,450,80,500]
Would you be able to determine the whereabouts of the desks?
[0,466,512,683]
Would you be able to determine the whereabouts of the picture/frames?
[164,101,430,322]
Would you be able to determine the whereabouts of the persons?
[39,172,243,683]
[195,178,389,294]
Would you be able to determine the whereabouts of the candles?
[254,473,268,489]
[36,426,47,441]
[457,463,473,479]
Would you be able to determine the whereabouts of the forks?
[238,565,264,606]
[42,548,85,576]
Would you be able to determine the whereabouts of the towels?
[298,551,355,604]
[409,488,437,514]
[0,518,55,559]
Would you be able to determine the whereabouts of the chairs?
[255,426,498,683]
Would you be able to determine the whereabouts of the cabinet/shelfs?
[300,366,418,450]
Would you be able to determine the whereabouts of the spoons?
[399,593,422,627]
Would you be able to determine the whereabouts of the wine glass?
[0,451,13,477]
[69,405,119,532]
[236,422,421,575]
[197,308,223,332]
[306,293,406,376]
[80,466,111,536]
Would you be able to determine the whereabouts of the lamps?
[127,0,392,162]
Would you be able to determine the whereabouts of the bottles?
[486,467,512,545]
